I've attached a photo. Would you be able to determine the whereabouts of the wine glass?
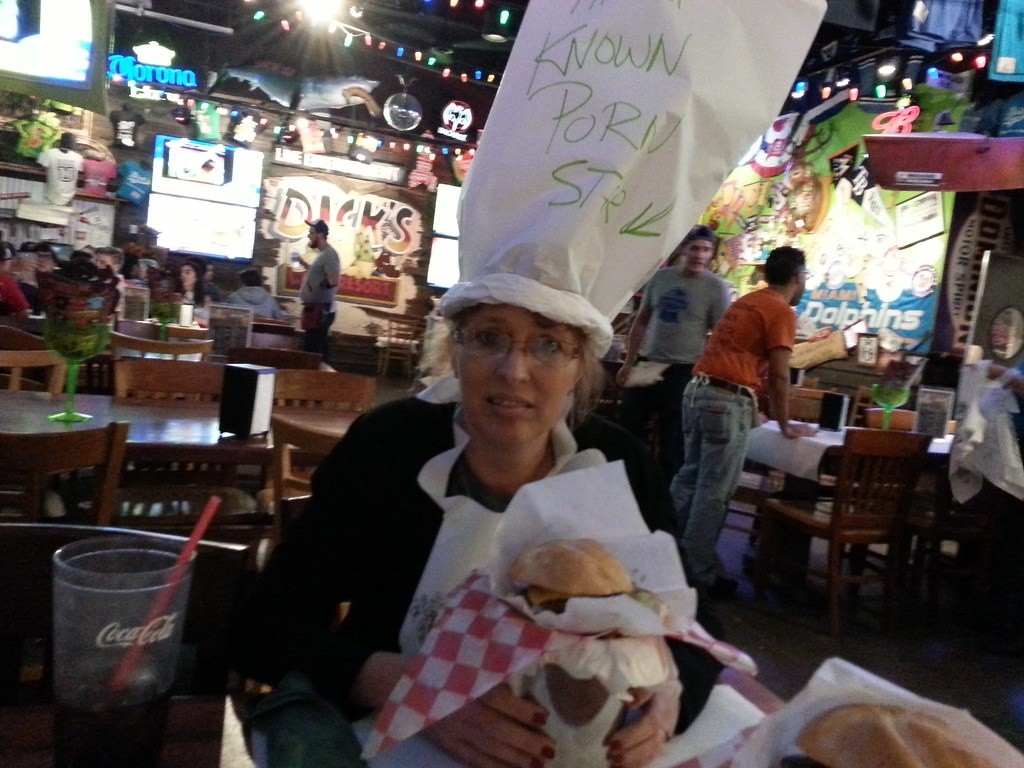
[872,383,911,430]
[43,315,111,422]
[149,303,181,341]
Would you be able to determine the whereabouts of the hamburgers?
[511,539,633,612]
[782,702,996,768]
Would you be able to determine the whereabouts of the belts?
[691,375,752,398]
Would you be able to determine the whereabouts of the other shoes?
[687,577,739,600]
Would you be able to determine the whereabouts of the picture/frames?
[856,333,879,369]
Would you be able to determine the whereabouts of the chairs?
[0,523,250,712]
[114,359,256,522]
[0,350,68,521]
[270,416,343,548]
[378,317,418,378]
[109,331,214,398]
[0,419,129,528]
[0,325,51,391]
[259,370,378,532]
[715,389,832,544]
[764,430,932,637]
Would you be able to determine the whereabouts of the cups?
[51,537,197,714]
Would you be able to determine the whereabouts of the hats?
[181,257,206,277]
[439,1,830,361]
[680,223,716,248]
[305,219,329,235]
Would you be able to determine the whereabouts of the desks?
[0,305,953,768]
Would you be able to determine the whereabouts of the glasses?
[800,269,813,280]
[452,324,583,367]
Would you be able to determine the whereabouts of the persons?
[218,234,677,767]
[293,219,339,359]
[223,270,283,317]
[662,246,818,647]
[615,226,727,529]
[0,235,222,331]
